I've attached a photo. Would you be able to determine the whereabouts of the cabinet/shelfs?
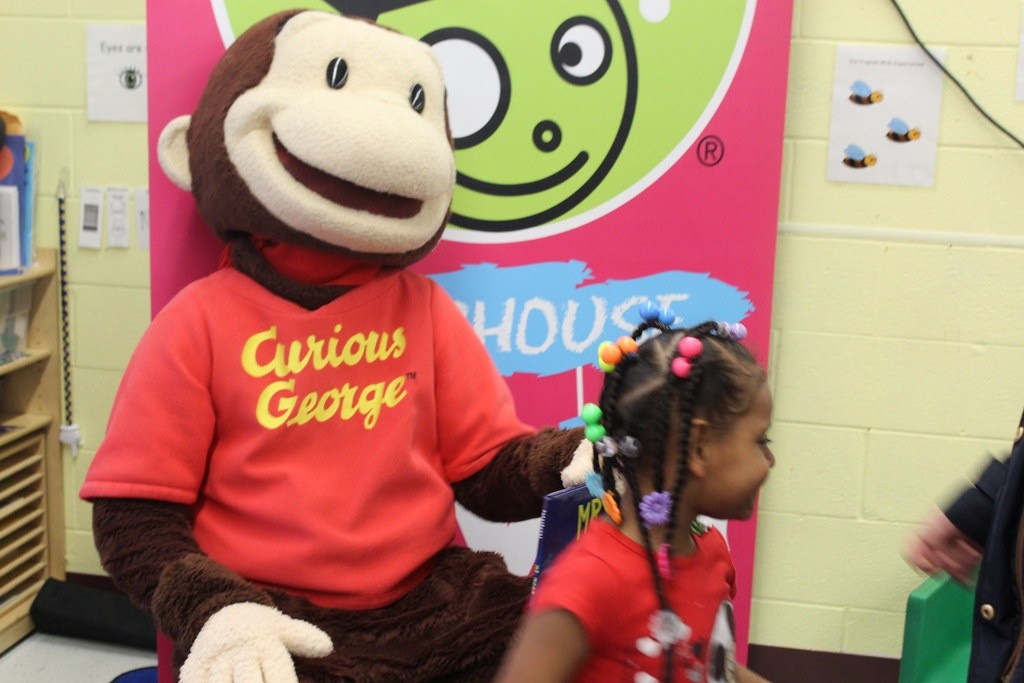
[0,243,64,657]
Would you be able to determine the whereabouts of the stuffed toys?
[81,9,602,683]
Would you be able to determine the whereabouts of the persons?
[912,408,1024,683]
[496,300,775,683]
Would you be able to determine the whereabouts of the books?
[531,482,602,594]
